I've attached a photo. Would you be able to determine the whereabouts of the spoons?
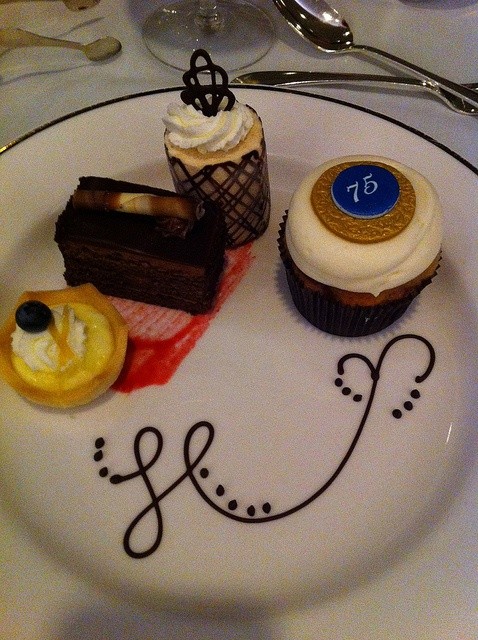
[272,1,478,108]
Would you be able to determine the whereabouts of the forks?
[231,69,478,115]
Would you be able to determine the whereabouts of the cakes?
[276,147,445,336]
[50,175,228,313]
[159,49,271,244]
[2,281,131,409]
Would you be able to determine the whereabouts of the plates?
[1,83,477,640]
